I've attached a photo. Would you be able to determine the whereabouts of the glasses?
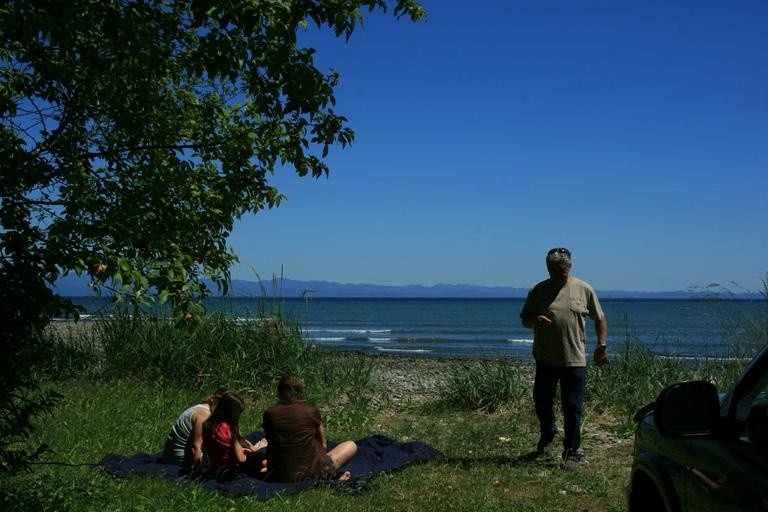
[548,247,570,256]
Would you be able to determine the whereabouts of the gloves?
[593,344,608,367]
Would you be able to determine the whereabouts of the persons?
[519,247,608,461]
[165,387,268,468]
[204,393,268,480]
[261,375,358,483]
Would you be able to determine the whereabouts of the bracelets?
[598,343,606,349]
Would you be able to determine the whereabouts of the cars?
[627,349,768,510]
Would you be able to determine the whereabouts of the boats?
[50,314,90,321]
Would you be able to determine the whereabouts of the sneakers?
[562,449,581,466]
[537,428,558,451]
